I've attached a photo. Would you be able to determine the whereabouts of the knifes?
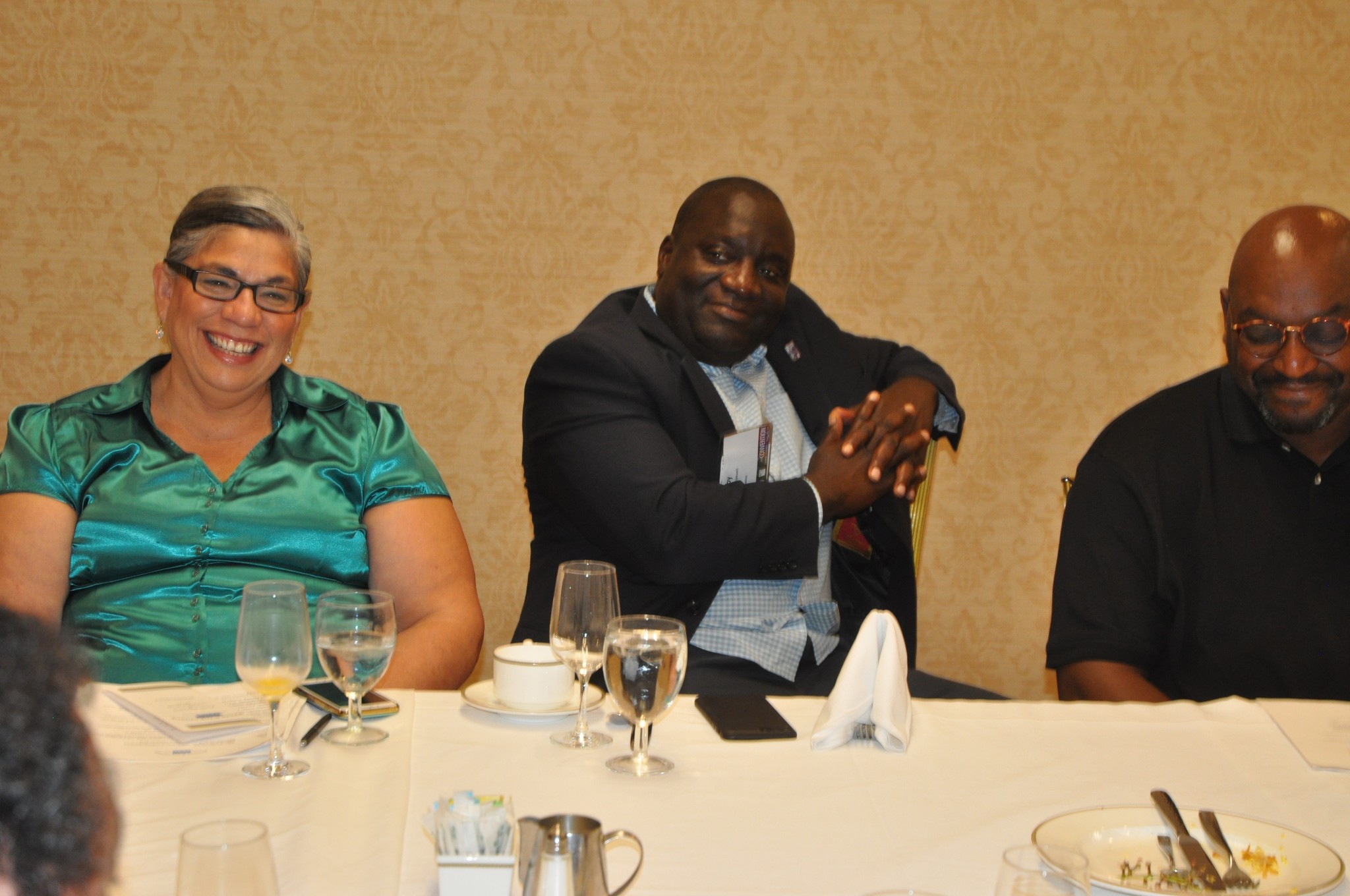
[1150,790,1226,894]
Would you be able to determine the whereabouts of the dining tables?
[0,685,1350,896]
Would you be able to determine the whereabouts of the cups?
[493,642,576,711]
[995,842,1092,896]
[434,855,514,896]
[174,818,279,895]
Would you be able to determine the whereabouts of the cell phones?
[294,683,399,720]
[693,695,797,741]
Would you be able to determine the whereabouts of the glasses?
[1228,301,1350,359]
[162,257,305,314]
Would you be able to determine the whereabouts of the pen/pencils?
[300,712,334,748]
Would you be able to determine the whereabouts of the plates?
[460,677,607,723]
[1030,805,1347,896]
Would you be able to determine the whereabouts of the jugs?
[510,812,645,896]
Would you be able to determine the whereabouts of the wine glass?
[234,579,311,781]
[315,588,396,747]
[549,560,622,751]
[602,613,687,778]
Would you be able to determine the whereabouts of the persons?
[1,185,487,691]
[1046,206,1350,700]
[521,177,964,691]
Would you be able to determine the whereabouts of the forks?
[1198,810,1260,890]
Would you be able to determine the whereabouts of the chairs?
[822,480,918,671]
